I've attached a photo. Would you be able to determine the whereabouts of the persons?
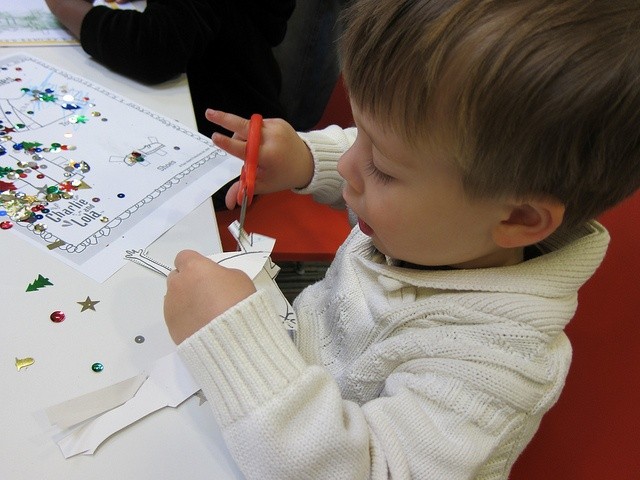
[163,2,639,480]
[45,0,345,209]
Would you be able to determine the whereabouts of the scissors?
[236,114,263,253]
